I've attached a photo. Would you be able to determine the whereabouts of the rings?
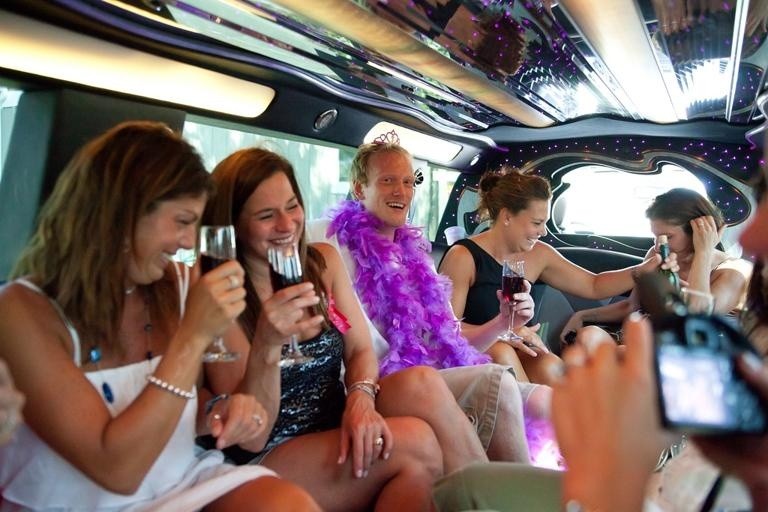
[251,415,261,425]
[698,220,704,226]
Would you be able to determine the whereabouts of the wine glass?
[266,245,314,369]
[192,225,243,365]
[499,260,528,343]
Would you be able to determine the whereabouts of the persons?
[429,259,767,510]
[200,147,446,512]
[0,119,325,512]
[326,144,536,466]
[558,187,753,348]
[538,171,768,511]
[436,169,680,389]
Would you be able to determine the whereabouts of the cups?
[443,226,466,246]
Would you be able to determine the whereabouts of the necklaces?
[86,295,152,417]
[122,284,136,294]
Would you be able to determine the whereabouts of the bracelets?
[557,492,586,512]
[144,375,196,401]
[348,379,381,399]
[202,393,230,417]
[630,265,640,285]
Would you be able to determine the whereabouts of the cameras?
[657,315,764,436]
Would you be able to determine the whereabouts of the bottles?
[654,231,678,299]
